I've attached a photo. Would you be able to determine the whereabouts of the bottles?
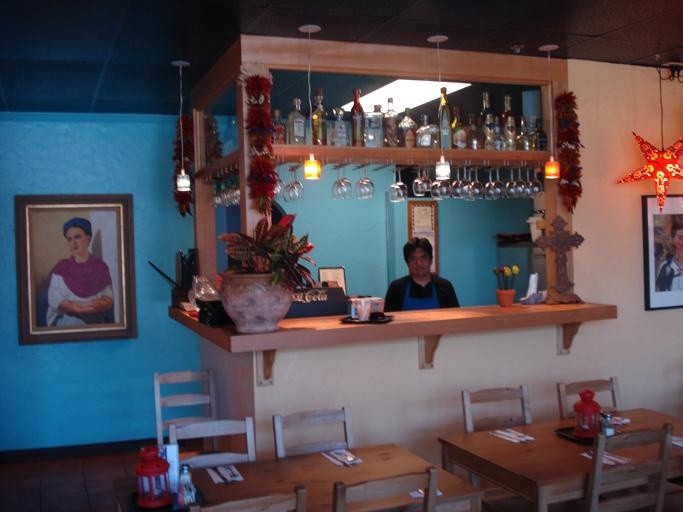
[601,411,614,438]
[176,463,198,506]
[273,86,542,151]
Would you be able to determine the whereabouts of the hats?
[64,219,91,236]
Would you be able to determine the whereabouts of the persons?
[655,224,682,291]
[43,215,114,326]
[382,238,460,313]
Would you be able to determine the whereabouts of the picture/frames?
[13,192,140,346]
[639,191,683,314]
[317,266,348,298]
[406,200,439,277]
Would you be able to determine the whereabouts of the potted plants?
[213,212,321,333]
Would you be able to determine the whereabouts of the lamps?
[296,24,322,180]
[168,59,192,194]
[612,48,682,211]
[538,43,561,180]
[425,34,454,180]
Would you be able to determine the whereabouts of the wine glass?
[271,161,546,203]
[203,165,244,208]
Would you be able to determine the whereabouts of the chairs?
[458,373,682,511]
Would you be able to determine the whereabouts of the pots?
[346,294,385,323]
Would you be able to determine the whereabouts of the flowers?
[493,264,519,290]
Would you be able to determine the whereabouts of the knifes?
[322,450,351,469]
[209,464,230,487]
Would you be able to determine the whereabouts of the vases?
[496,289,516,307]
